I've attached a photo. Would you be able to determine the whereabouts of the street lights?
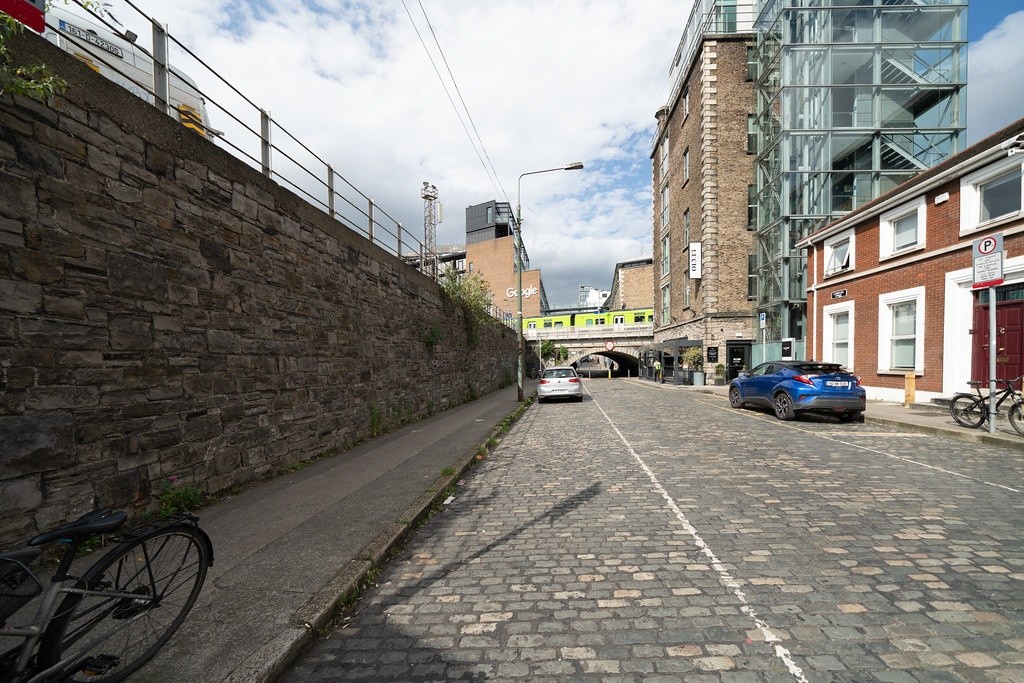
[514,161,584,403]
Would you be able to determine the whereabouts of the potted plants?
[716,364,725,385]
[682,346,702,384]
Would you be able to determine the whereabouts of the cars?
[537,366,584,402]
[728,361,866,423]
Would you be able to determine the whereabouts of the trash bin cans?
[693,371,704,386]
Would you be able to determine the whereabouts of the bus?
[0,0,224,146]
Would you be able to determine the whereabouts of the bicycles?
[949,376,1024,436]
[0,505,216,683]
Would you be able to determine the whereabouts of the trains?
[519,306,654,328]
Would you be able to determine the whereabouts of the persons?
[611,361,614,370]
[653,359,661,382]
[573,362,577,370]
